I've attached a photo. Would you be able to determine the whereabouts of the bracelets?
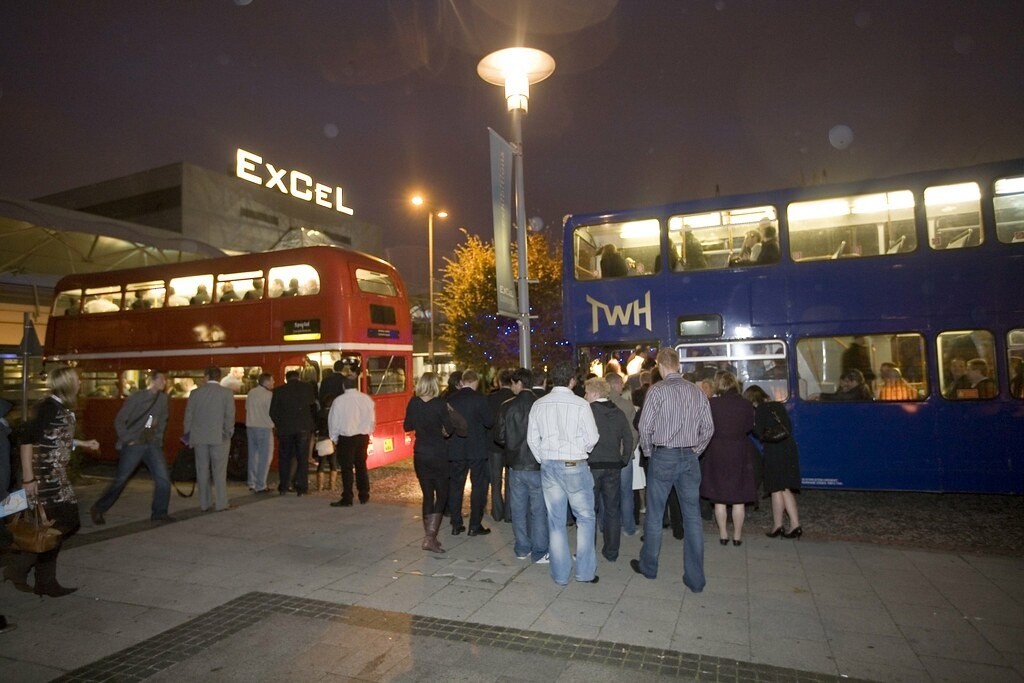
[22,479,36,483]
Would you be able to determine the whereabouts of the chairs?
[749,228,974,264]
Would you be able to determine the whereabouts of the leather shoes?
[468,526,491,536]
[451,525,465,535]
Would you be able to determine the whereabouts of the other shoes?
[280,486,309,497]
[91,506,106,526]
[536,553,551,564]
[250,487,274,493]
[330,498,353,507]
[733,539,742,546]
[720,537,729,545]
[588,576,600,583]
[517,551,532,560]
[683,576,705,593]
[631,559,656,579]
[359,495,370,503]
[0,622,17,634]
[152,512,173,520]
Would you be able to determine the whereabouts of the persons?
[245,371,315,496]
[301,356,374,507]
[630,347,715,593]
[808,330,1024,403]
[64,278,319,316]
[403,366,554,563]
[527,361,600,586]
[0,366,100,633]
[670,345,802,546]
[115,366,263,397]
[183,365,236,513]
[573,345,663,561]
[90,370,175,526]
[595,217,779,278]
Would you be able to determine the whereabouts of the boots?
[32,545,78,598]
[330,471,338,491]
[4,551,39,593]
[317,472,325,492]
[422,513,447,553]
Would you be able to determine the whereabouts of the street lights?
[476,46,556,368]
[413,196,448,371]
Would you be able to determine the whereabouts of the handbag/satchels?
[758,411,790,442]
[172,447,198,483]
[7,496,61,552]
[316,439,334,456]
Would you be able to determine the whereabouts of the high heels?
[766,525,803,540]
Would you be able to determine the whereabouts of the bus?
[563,156,1024,495]
[41,244,418,480]
